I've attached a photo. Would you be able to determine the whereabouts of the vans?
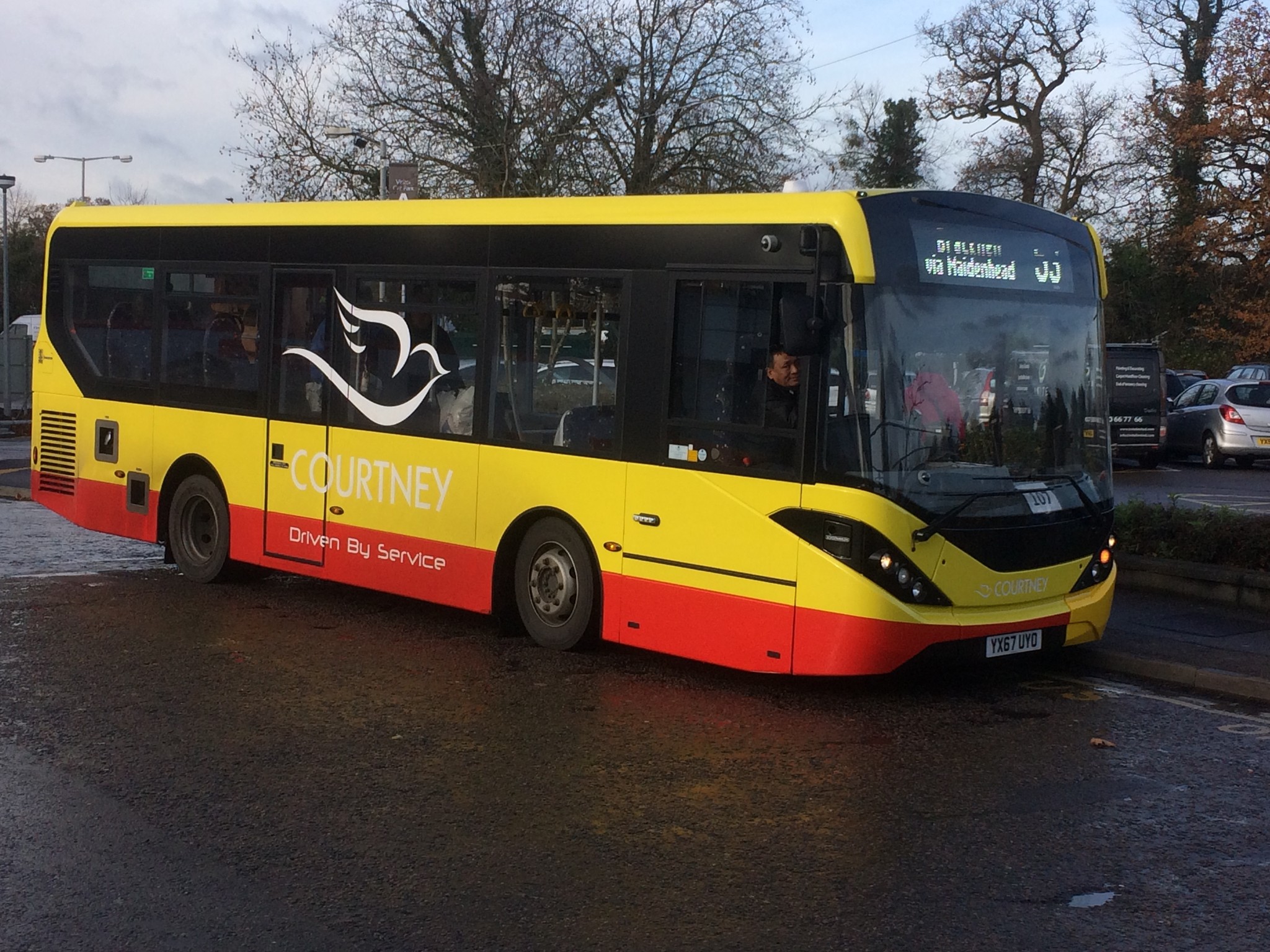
[988,340,1169,469]
[0,314,42,348]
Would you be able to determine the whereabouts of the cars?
[1156,378,1270,470]
[825,361,999,433]
[1166,363,1270,407]
[440,355,615,412]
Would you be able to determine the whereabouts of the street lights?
[323,127,389,200]
[0,174,17,418]
[34,154,134,201]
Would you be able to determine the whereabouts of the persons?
[744,349,800,465]
[905,364,965,445]
[308,318,325,390]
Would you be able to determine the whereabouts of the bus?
[26,178,1119,681]
